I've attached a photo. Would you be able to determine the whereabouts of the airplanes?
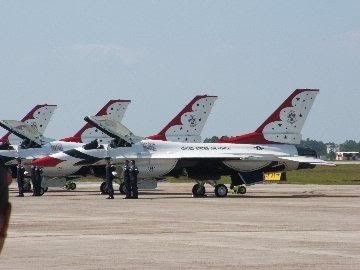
[6,95,219,195]
[1,104,57,148]
[0,99,131,189]
[29,89,337,197]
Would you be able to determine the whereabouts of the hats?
[105,156,111,160]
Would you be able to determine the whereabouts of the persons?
[103,157,115,199]
[34,166,43,196]
[122,160,131,199]
[30,159,36,196]
[129,160,139,199]
[16,159,26,197]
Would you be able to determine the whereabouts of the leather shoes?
[109,197,114,199]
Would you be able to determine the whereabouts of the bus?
[336,151,360,160]
